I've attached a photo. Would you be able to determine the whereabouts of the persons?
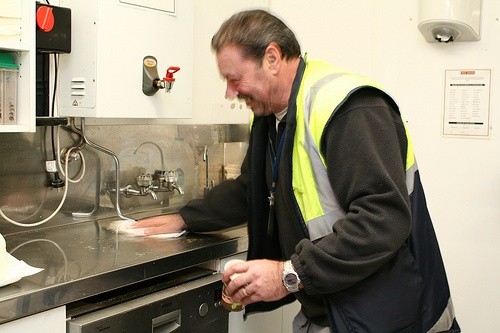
[130,9,464,333]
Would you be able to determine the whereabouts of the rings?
[243,289,249,296]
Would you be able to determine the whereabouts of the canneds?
[221,259,246,312]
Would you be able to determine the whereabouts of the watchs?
[282,260,301,292]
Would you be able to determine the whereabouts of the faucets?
[202,144,210,199]
[155,65,180,92]
[152,170,185,195]
[119,184,158,200]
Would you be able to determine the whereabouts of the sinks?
[129,202,188,221]
